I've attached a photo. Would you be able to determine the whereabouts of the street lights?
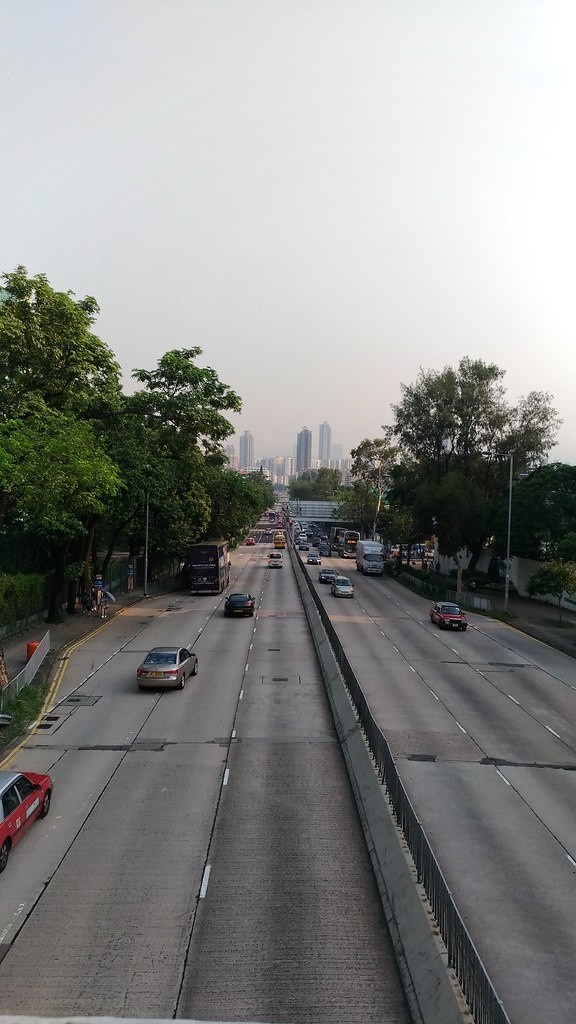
[479,451,514,611]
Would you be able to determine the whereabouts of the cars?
[137,647,198,691]
[245,538,254,546]
[429,600,467,632]
[318,568,339,584]
[329,575,354,598]
[264,508,285,527]
[0,769,54,872]
[221,592,256,617]
[292,517,333,551]
[318,544,331,557]
[306,553,321,566]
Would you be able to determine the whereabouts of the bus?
[330,527,348,551]
[186,540,232,596]
[387,542,425,561]
[337,530,360,559]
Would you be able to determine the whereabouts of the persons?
[421,559,441,572]
[82,588,110,619]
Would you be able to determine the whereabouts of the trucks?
[273,532,286,549]
[355,540,384,576]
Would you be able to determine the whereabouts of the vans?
[266,551,283,568]
